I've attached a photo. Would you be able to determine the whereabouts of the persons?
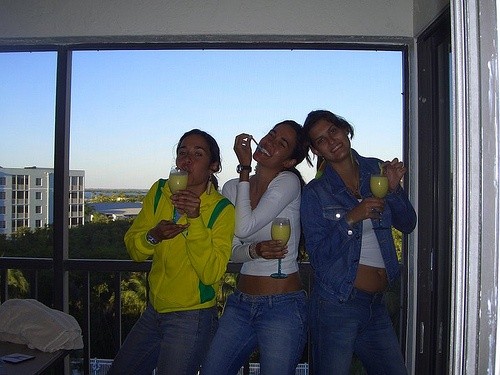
[200,119,313,375]
[105,129,234,375]
[299,110,417,375]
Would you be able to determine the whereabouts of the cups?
[169,169,188,195]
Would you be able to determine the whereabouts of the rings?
[371,208,374,214]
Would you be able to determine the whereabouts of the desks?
[0,341,74,375]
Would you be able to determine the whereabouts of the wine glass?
[271,217,291,279]
[371,173,390,230]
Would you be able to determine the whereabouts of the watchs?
[236,164,252,173]
[147,229,162,245]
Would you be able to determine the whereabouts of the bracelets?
[250,241,262,259]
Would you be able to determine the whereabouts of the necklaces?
[348,181,361,199]
